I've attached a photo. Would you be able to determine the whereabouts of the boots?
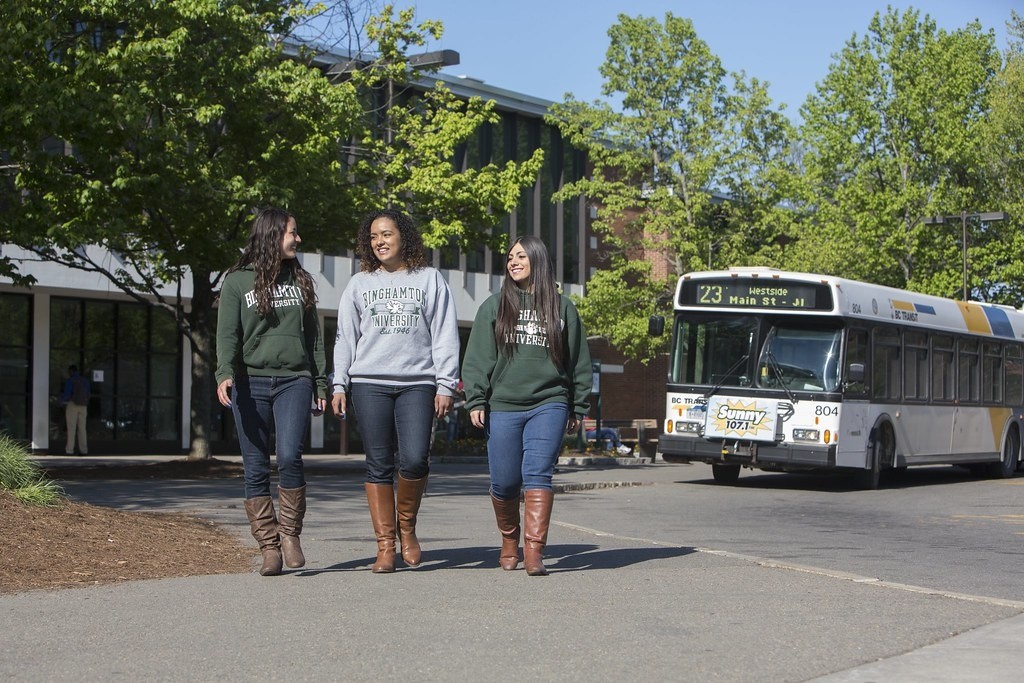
[244,496,283,575]
[276,481,307,568]
[489,486,520,570]
[396,470,430,567]
[364,482,396,573]
[522,489,554,575]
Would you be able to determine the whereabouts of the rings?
[445,410,449,413]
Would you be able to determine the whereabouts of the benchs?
[580,418,658,454]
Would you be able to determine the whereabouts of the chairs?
[848,362,867,394]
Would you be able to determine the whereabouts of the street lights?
[923,210,1010,300]
[323,50,461,207]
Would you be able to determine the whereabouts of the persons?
[332,210,461,572]
[567,426,632,457]
[60,365,93,456]
[214,208,329,577]
[462,236,593,576]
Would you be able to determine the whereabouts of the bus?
[648,266,1023,492]
